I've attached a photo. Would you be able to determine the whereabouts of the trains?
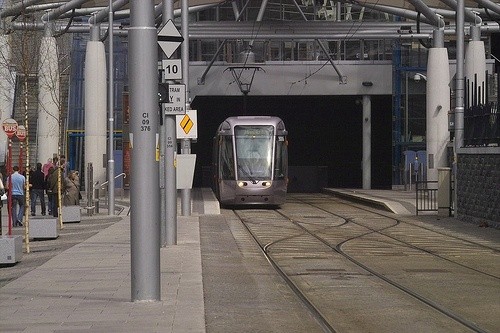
[208,115,289,209]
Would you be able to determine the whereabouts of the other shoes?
[31,211,35,215]
[48,209,52,214]
[17,219,23,226]
[42,212,45,215]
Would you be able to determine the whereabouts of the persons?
[22,153,81,217]
[6,166,27,227]
[0,172,5,236]
